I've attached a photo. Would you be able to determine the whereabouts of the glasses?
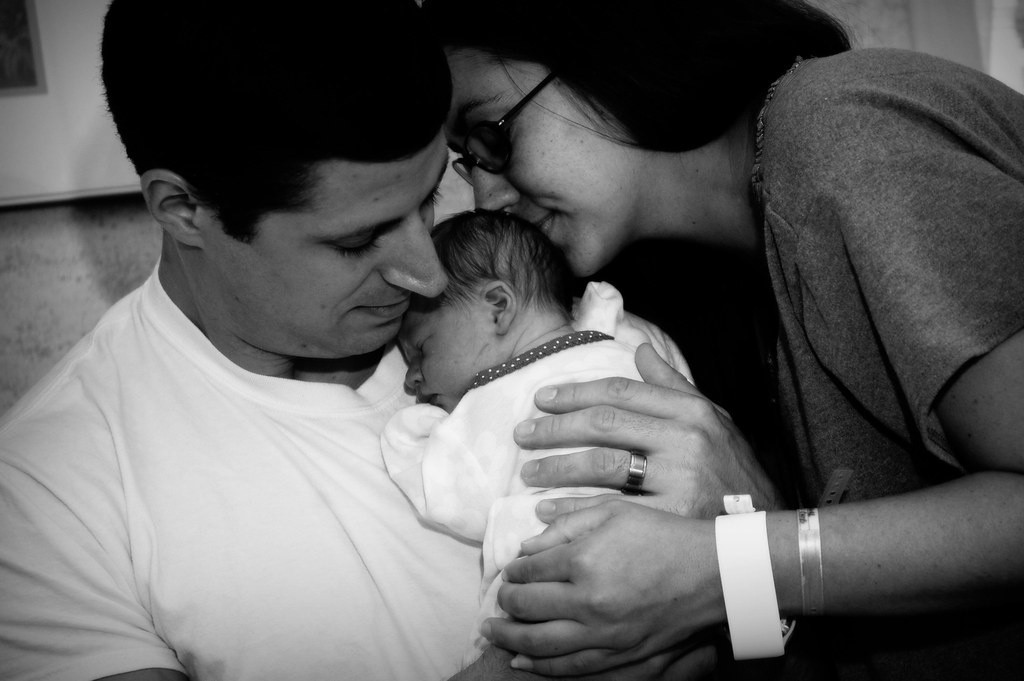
[453,69,560,189]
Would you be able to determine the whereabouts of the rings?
[621,450,646,494]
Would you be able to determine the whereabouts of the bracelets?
[798,507,823,619]
[715,494,795,660]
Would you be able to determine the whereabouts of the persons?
[0,0,789,681]
[438,0,1024,681]
[380,206,697,671]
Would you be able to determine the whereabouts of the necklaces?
[750,56,802,203]
